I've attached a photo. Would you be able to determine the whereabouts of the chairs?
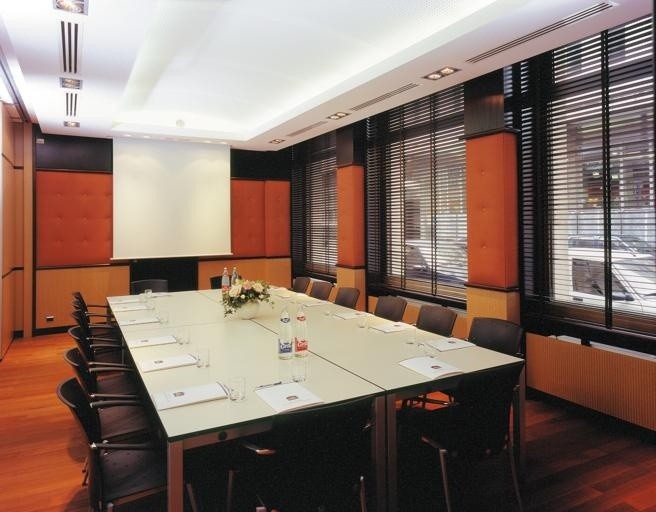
[375,296,408,323]
[309,282,334,301]
[230,396,375,512]
[469,317,525,358]
[416,304,458,337]
[55,281,213,510]
[287,397,376,478]
[396,359,531,510]
[336,286,361,310]
[397,360,526,478]
[293,277,310,294]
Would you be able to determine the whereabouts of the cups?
[137,290,211,370]
[228,375,246,400]
[407,327,416,344]
[319,304,374,328]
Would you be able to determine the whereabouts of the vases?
[236,300,260,318]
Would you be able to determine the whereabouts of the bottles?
[294,303,308,383]
[221,267,230,294]
[232,266,237,286]
[278,302,293,383]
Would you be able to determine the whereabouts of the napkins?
[284,288,295,299]
[371,321,416,333]
[285,381,323,411]
[427,336,475,352]
[337,311,375,320]
[304,301,330,307]
[398,356,464,379]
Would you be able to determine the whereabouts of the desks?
[198,274,529,512]
[98,284,389,512]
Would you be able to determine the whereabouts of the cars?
[568,232,656,258]
[400,235,467,286]
[567,247,655,317]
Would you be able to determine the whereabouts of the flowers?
[215,274,274,312]
